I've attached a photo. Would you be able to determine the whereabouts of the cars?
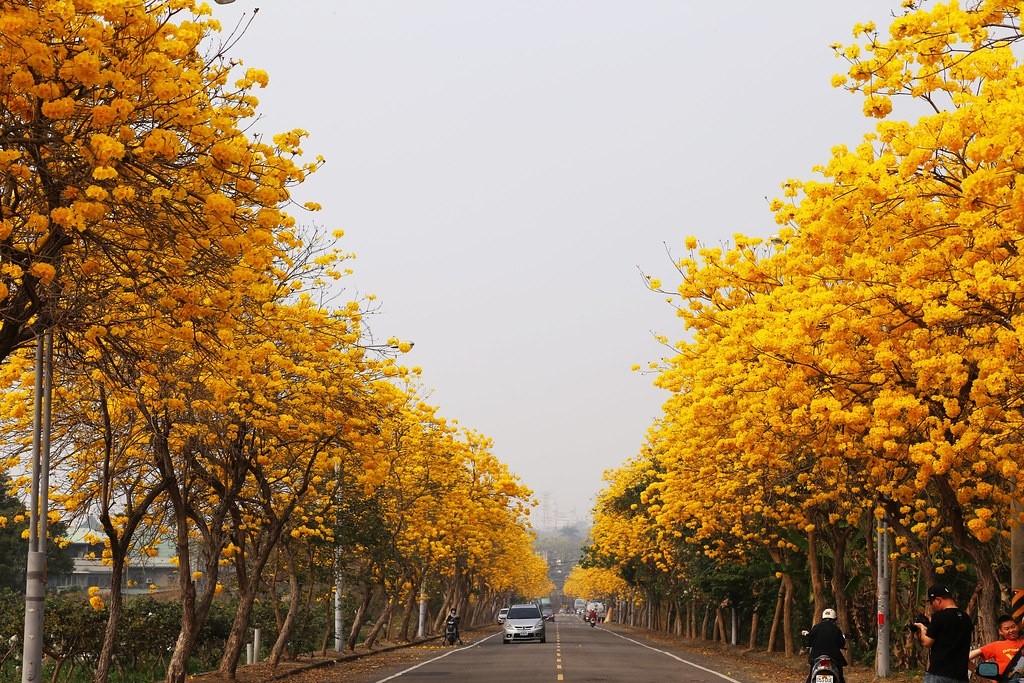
[577,606,585,619]
[977,642,1024,683]
[497,607,509,624]
[541,607,555,622]
[499,603,548,643]
[559,608,567,614]
[567,609,575,614]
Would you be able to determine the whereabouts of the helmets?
[591,609,594,612]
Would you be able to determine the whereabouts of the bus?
[533,597,553,611]
[574,599,585,611]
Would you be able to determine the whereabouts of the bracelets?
[920,628,927,631]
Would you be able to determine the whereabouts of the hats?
[822,609,836,619]
[926,584,951,602]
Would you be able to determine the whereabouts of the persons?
[913,584,974,683]
[801,608,848,683]
[588,609,606,623]
[968,614,1024,683]
[442,608,464,645]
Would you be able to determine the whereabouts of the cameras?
[910,614,931,634]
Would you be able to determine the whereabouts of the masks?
[452,611,455,614]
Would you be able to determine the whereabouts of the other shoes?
[460,641,463,645]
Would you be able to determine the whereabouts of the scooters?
[803,630,844,683]
[590,618,596,627]
[445,619,459,645]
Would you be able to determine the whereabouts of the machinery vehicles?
[585,599,606,623]
